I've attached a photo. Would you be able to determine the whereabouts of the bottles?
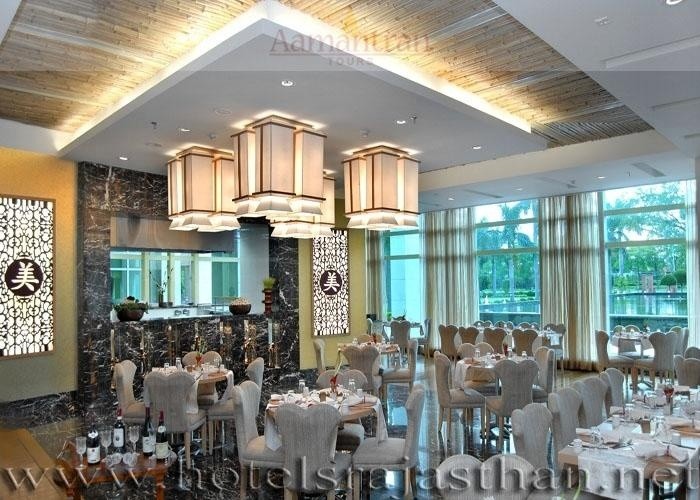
[142,406,155,457]
[113,410,124,455]
[156,409,169,463]
[87,415,100,467]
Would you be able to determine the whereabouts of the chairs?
[111,316,699,499]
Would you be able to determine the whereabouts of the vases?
[229,304,252,316]
[158,295,163,308]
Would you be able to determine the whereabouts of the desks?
[53,433,177,497]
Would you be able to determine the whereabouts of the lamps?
[340,141,425,235]
[163,145,244,237]
[227,111,327,219]
[267,171,339,242]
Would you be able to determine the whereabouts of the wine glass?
[100,425,158,468]
[590,427,603,460]
[664,379,674,417]
[75,438,87,469]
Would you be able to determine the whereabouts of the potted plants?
[260,276,278,314]
[113,302,148,321]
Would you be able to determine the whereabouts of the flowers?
[149,266,175,294]
[231,296,249,305]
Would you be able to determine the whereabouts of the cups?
[159,354,228,383]
[285,379,363,414]
[573,439,583,455]
[472,350,529,367]
[611,385,700,451]
